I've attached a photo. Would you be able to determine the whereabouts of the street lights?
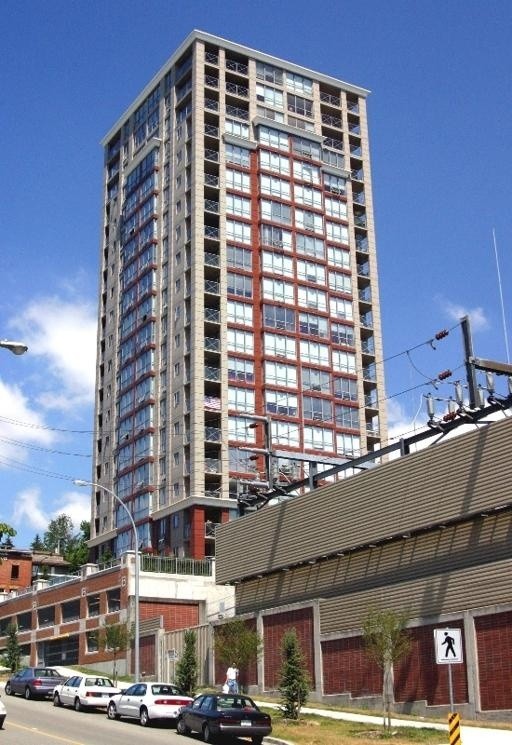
[75,478,141,687]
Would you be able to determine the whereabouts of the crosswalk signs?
[434,627,463,664]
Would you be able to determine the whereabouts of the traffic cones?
[448,712,461,745]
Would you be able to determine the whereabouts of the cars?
[5,666,70,701]
[176,693,272,744]
[107,680,195,727]
[52,674,123,711]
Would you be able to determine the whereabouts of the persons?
[0,695,7,731]
[225,662,240,695]
